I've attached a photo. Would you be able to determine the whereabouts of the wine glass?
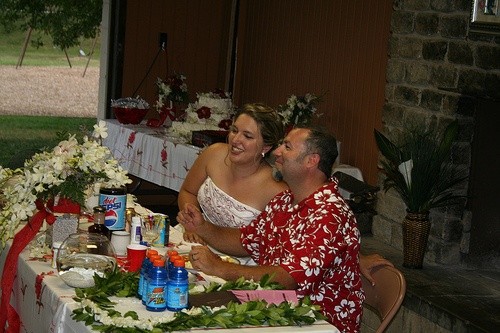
[139,215,165,247]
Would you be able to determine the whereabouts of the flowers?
[147,71,188,125]
[267,94,326,146]
[66,241,330,333]
[0,121,133,259]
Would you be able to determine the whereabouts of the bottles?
[56,233,117,289]
[136,250,189,312]
[87,206,111,256]
[129,217,142,244]
[98,178,127,238]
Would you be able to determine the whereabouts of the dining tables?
[0,196,342,333]
[98,116,364,202]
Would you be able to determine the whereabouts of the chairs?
[361,266,406,333]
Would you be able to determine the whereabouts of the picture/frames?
[469,0,500,26]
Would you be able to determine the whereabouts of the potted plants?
[373,114,470,269]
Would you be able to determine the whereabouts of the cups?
[111,230,130,258]
[126,244,148,273]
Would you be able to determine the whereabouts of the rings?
[191,255,194,259]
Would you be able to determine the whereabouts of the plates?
[166,252,241,273]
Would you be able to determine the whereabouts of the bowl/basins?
[112,107,150,124]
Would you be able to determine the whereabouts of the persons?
[177,125,366,333]
[166,104,289,263]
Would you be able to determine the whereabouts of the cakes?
[172,88,234,147]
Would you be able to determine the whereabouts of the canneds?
[152,214,171,248]
